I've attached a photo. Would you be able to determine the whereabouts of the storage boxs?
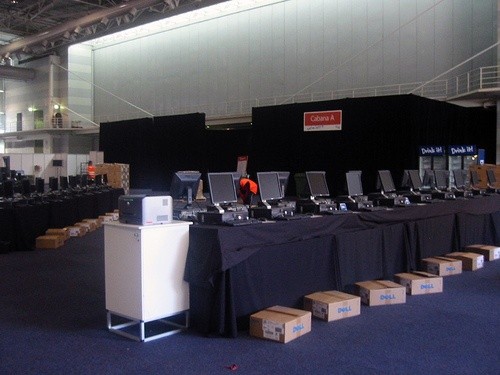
[35,163,130,248]
[249,242,500,343]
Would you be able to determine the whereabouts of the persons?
[87,161,96,180]
[237,177,258,205]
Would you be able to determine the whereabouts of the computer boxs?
[171,186,495,223]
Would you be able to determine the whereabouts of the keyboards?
[361,206,387,212]
[280,215,310,220]
[225,219,262,226]
[325,209,353,215]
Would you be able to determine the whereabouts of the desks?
[104,221,193,342]
[0,185,125,255]
[186,190,500,338]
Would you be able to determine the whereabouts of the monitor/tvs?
[206,173,237,205]
[232,172,246,198]
[0,172,108,199]
[271,171,291,197]
[173,171,199,199]
[258,172,284,202]
[293,167,497,198]
